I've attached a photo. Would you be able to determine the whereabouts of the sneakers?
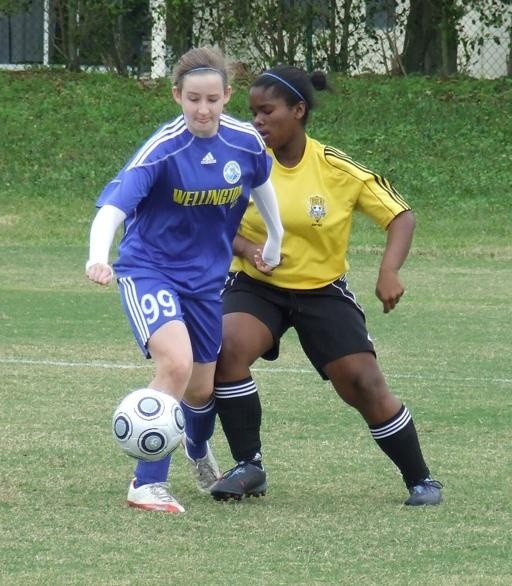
[180,431,222,494]
[210,451,269,502]
[405,475,444,506]
[127,478,186,515]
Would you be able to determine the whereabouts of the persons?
[209,66,443,508]
[85,46,283,515]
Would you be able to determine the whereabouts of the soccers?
[112,389,185,463]
